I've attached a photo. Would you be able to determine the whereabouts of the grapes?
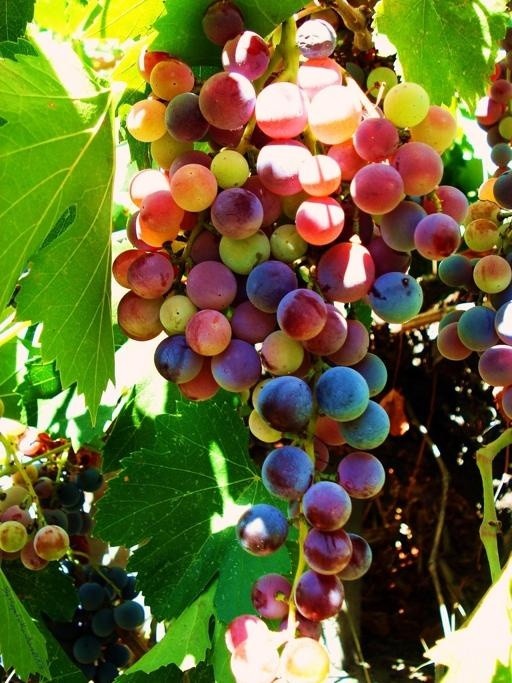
[0,0,512,683]
